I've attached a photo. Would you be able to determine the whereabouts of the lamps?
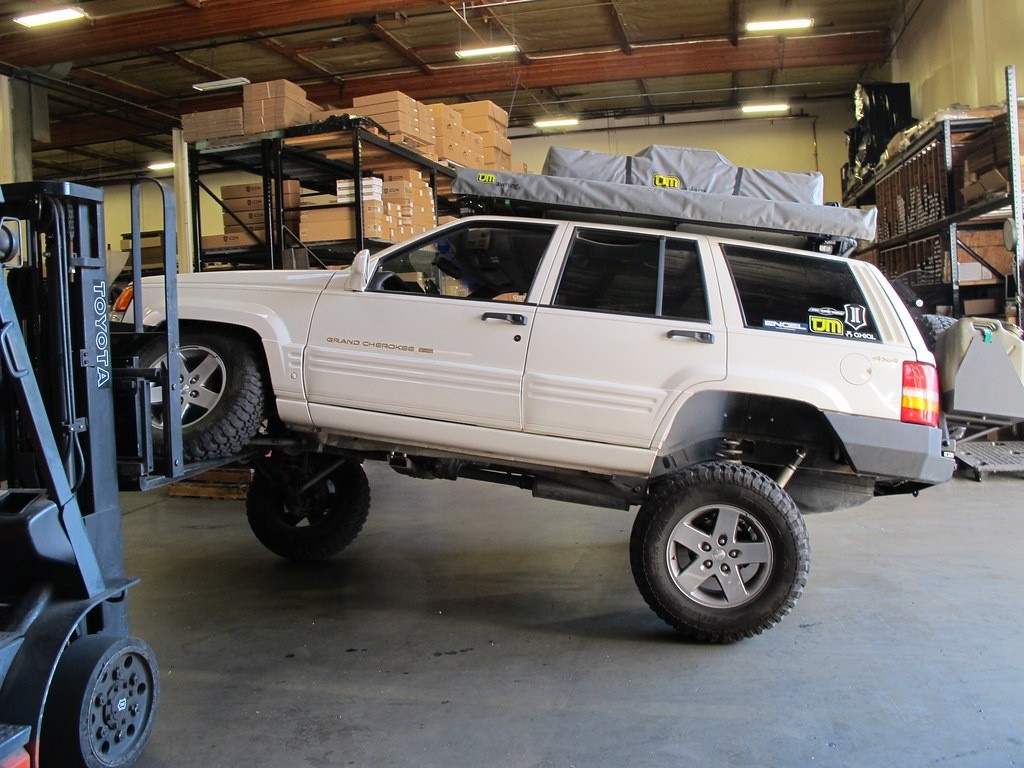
[532,118,580,127]
[12,6,88,28]
[746,17,815,32]
[455,44,520,59]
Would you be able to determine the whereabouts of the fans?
[891,270,951,318]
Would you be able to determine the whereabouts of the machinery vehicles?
[0,182,258,768]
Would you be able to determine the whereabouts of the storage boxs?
[849,102,1024,316]
[180,80,527,303]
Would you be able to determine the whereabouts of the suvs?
[107,186,952,646]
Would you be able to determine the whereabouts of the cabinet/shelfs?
[840,66,1024,450]
[188,138,357,273]
[274,126,457,295]
